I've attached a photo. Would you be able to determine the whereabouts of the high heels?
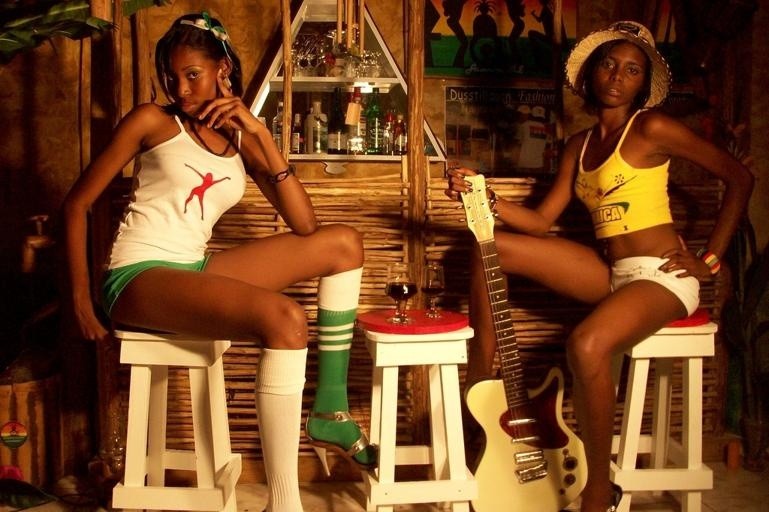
[606,481,623,512]
[305,409,380,476]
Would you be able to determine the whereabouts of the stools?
[610,308,719,512]
[356,309,479,512]
[113,328,242,512]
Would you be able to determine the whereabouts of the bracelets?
[696,247,721,275]
[268,165,295,185]
[489,191,499,211]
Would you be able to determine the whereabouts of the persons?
[444,20,756,511]
[58,12,379,512]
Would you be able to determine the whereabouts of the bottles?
[272,98,284,153]
[327,87,348,154]
[394,115,408,156]
[347,87,367,154]
[383,113,395,155]
[365,87,384,153]
[292,113,304,153]
[305,101,328,154]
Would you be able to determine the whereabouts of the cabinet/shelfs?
[248,1,448,162]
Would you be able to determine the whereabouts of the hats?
[564,21,673,114]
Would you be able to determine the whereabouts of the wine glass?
[420,265,445,318]
[383,262,418,327]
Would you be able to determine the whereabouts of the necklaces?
[189,118,235,157]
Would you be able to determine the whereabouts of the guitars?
[451,174,588,512]
